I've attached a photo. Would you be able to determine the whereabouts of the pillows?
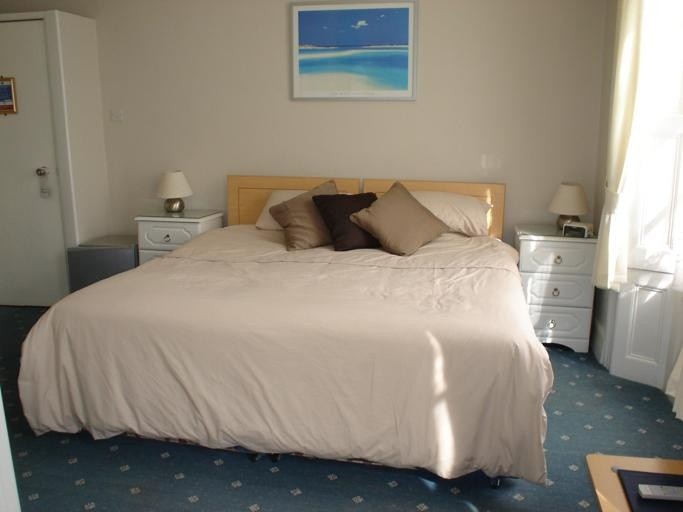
[256,179,494,255]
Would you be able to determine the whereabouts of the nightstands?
[514,224,598,353]
[134,210,224,266]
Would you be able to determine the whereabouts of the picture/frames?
[290,0,419,100]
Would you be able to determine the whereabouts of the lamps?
[548,183,589,228]
[156,172,193,213]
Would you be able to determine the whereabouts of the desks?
[586,453,683,512]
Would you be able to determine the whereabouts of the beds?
[17,175,555,488]
